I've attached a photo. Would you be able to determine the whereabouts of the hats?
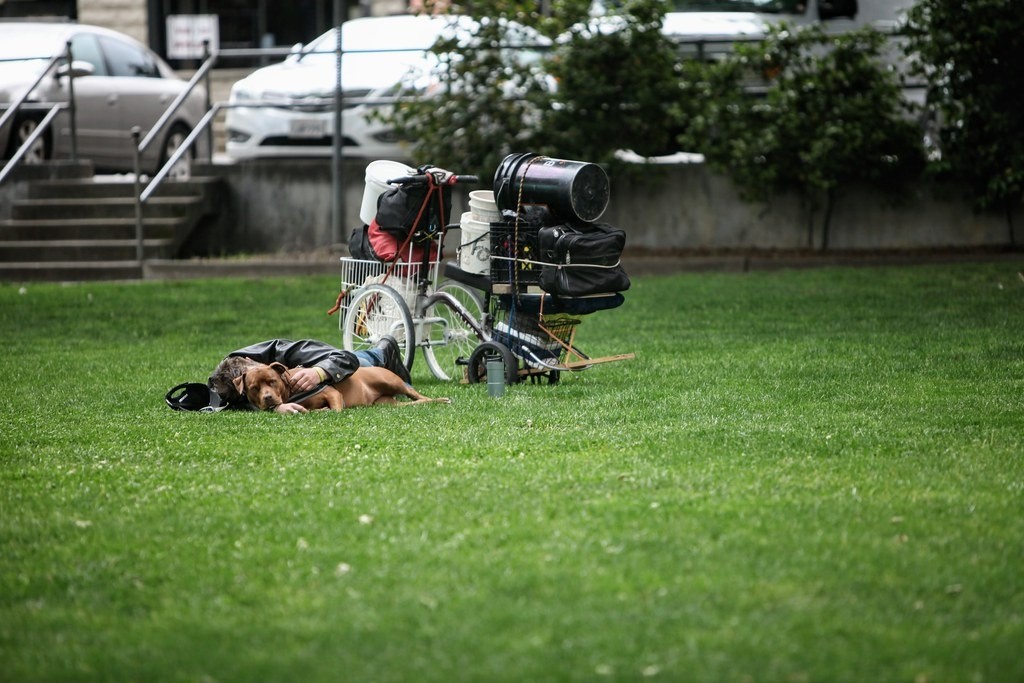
[165,382,220,413]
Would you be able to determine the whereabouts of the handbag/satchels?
[348,174,453,279]
[537,222,631,296]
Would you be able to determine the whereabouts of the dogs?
[232,361,451,414]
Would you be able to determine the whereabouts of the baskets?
[490,296,581,372]
[338,256,440,338]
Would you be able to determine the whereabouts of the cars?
[0,22,210,181]
[224,14,554,177]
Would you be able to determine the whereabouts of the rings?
[295,410,298,413]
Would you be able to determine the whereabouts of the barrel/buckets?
[456,190,502,276]
[492,152,611,222]
[360,160,419,227]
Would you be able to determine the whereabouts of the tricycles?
[338,165,593,386]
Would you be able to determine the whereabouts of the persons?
[207,335,413,413]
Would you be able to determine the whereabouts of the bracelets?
[317,369,324,382]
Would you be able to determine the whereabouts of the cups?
[486,362,505,398]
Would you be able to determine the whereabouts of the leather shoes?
[376,335,412,385]
